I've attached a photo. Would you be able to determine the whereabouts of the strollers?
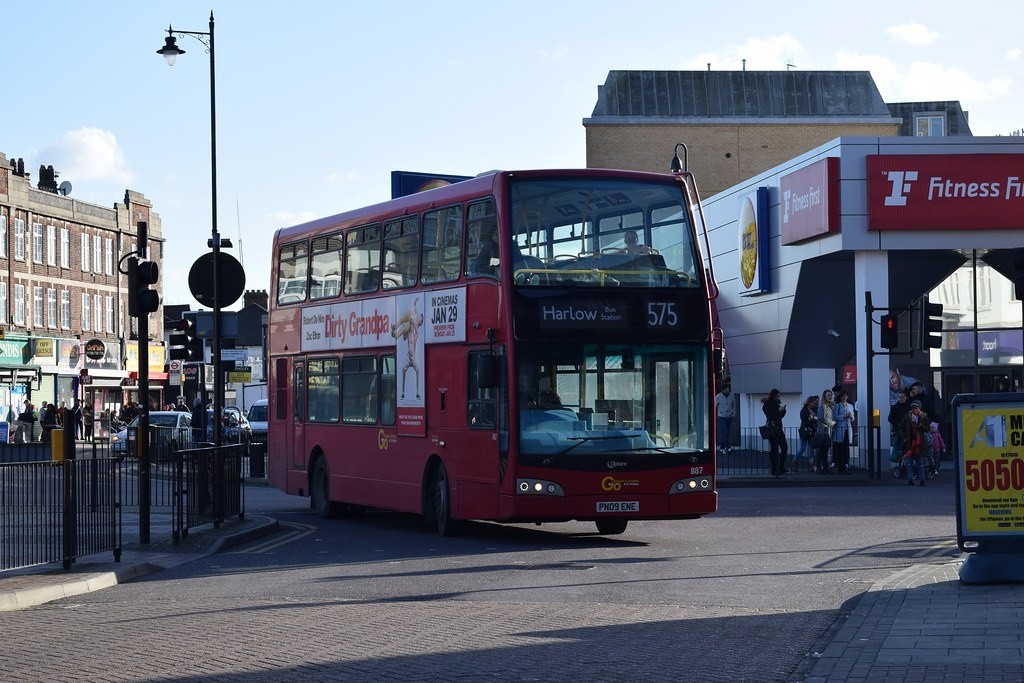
[892,426,937,481]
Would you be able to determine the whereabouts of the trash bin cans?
[249,441,264,478]
[41,423,61,441]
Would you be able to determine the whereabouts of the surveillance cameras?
[670,156,682,174]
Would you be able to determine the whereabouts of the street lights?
[155,8,223,445]
[670,142,689,185]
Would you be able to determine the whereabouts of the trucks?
[234,382,269,417]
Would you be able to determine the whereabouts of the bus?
[265,166,728,541]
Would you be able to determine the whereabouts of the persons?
[888,383,946,487]
[189,398,209,445]
[400,319,422,399]
[23,400,38,442]
[762,389,790,474]
[82,402,92,443]
[163,401,191,413]
[791,386,855,472]
[715,384,736,454]
[39,402,56,441]
[476,225,523,275]
[1000,379,1011,394]
[620,231,652,255]
[58,402,68,427]
[101,402,139,434]
[73,399,85,440]
[528,372,562,408]
[887,365,928,407]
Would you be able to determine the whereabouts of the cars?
[110,412,192,463]
[205,408,252,457]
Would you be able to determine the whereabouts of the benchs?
[99,429,107,436]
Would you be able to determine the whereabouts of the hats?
[929,422,938,432]
[911,401,921,408]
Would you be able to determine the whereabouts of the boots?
[792,457,799,473]
[808,463,815,472]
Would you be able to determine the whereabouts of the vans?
[247,400,268,445]
[370,373,395,425]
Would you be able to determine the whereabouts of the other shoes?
[832,467,836,474]
[720,447,732,453]
[919,480,925,486]
[908,480,915,486]
[837,464,851,474]
[934,469,939,474]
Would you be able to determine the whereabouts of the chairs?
[323,248,660,302]
[343,415,377,423]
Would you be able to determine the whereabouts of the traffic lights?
[880,314,899,348]
[179,310,206,364]
[921,294,944,353]
[127,257,159,318]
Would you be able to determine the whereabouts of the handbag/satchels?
[812,423,832,447]
[798,424,815,439]
[759,418,780,439]
